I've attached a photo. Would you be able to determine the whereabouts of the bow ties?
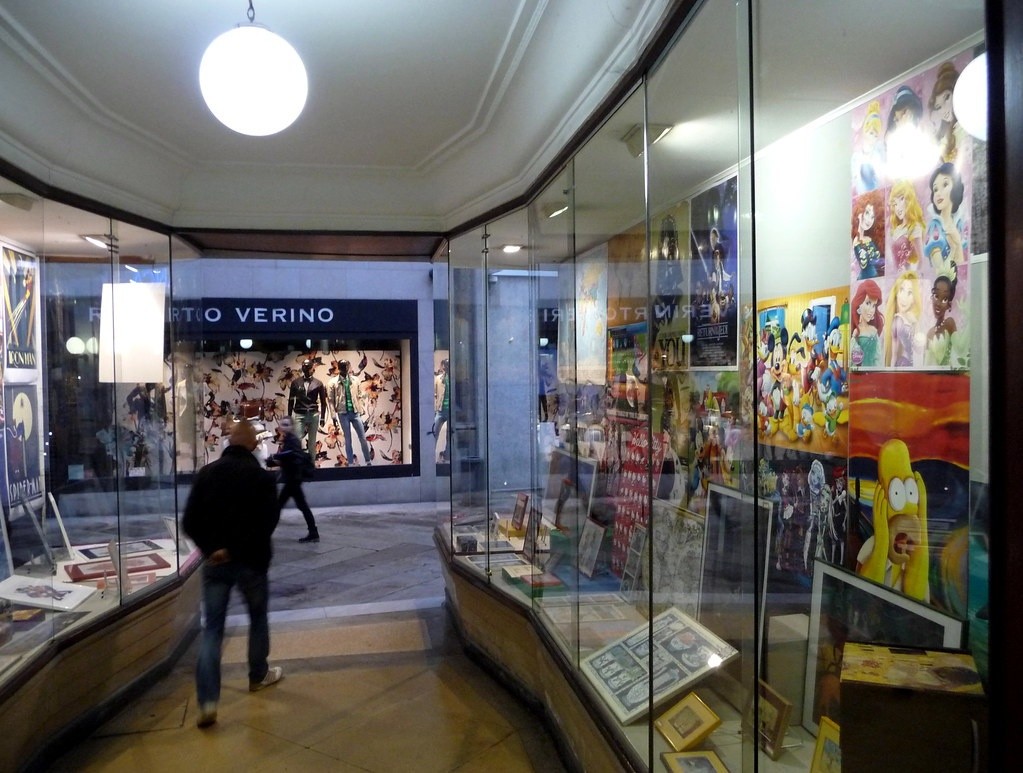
[302,378,312,382]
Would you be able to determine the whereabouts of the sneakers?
[248,666,283,691]
[196,701,218,730]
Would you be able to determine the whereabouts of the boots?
[298,526,320,542]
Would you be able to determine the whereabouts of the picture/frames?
[522,508,543,565]
[634,498,705,622]
[654,692,722,753]
[660,750,731,773]
[799,557,969,739]
[696,482,779,714]
[511,492,529,531]
[740,676,793,761]
[164,515,191,553]
[543,445,599,544]
[809,714,842,773]
[579,605,740,726]
[572,518,607,578]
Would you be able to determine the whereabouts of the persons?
[288,359,326,466]
[263,417,320,542]
[327,359,372,466]
[435,360,449,461]
[180,424,282,726]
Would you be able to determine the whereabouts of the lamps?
[66,337,88,365]
[198,0,308,136]
[622,124,675,157]
[542,203,569,219]
